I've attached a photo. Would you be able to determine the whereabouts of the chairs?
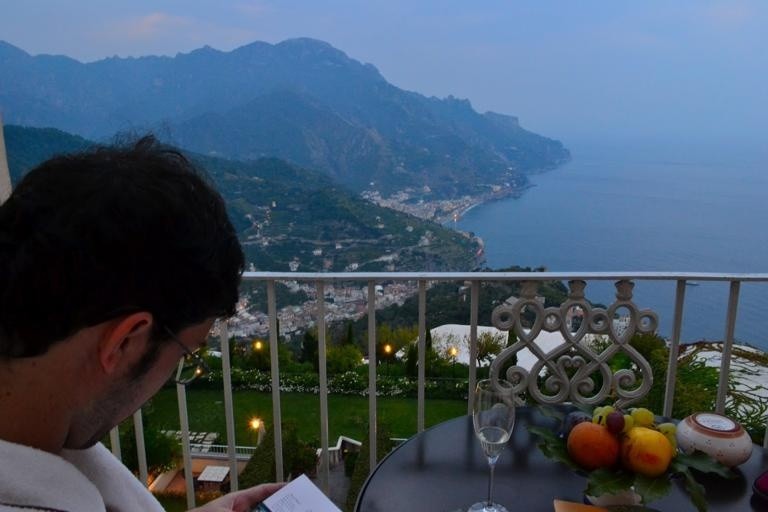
[181,468,204,492]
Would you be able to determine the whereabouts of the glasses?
[157,323,210,385]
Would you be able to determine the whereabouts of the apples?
[566,415,620,469]
[621,426,673,479]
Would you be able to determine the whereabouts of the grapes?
[593,405,677,458]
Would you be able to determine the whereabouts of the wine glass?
[467,379,515,512]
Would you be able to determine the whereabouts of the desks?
[197,465,230,488]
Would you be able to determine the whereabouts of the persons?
[0,129,290,512]
